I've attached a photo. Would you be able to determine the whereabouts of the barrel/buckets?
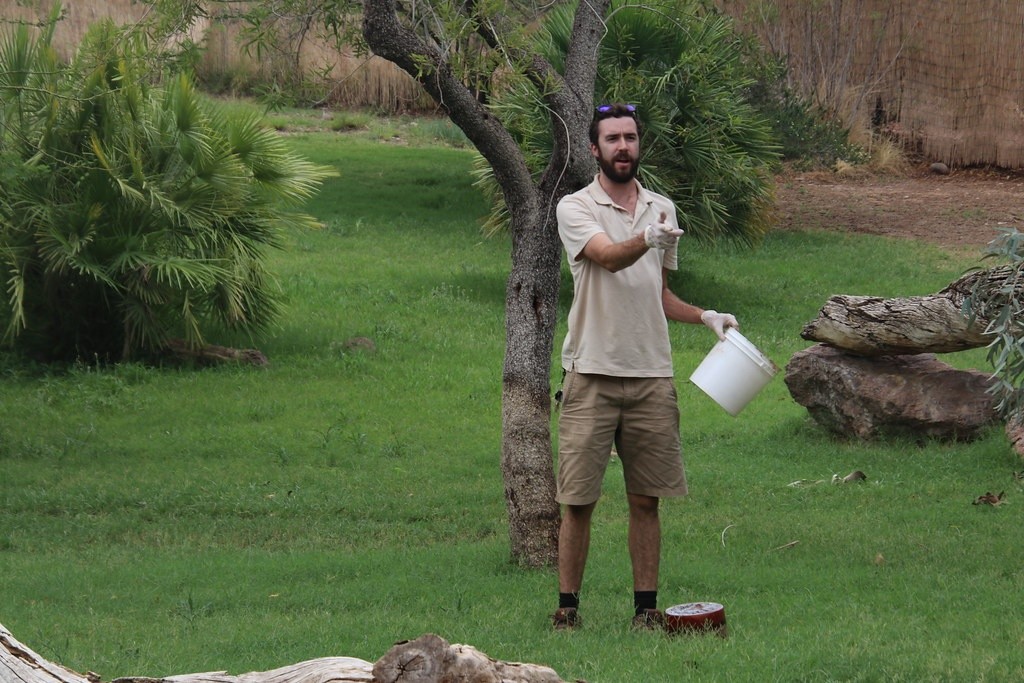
[689,327,776,416]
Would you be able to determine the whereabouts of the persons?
[548,105,739,634]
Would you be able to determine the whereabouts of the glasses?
[593,103,638,124]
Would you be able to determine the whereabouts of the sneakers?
[631,606,674,634]
[549,607,582,630]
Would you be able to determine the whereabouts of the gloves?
[644,211,684,270]
[701,308,740,341]
